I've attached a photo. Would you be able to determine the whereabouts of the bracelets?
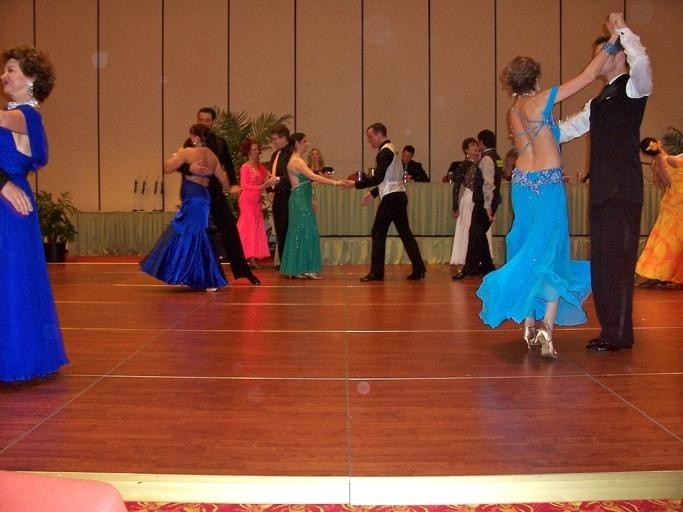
[603,41,615,53]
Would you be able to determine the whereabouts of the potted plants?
[200,105,292,263]
[32,188,72,263]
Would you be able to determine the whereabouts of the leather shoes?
[246,274,259,285]
[408,270,423,279]
[589,338,598,344]
[453,271,463,280]
[359,273,383,282]
[585,343,632,352]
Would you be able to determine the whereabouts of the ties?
[271,150,281,190]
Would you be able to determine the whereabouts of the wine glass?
[447,172,454,184]
[402,164,408,179]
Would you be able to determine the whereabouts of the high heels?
[304,273,322,280]
[638,279,667,288]
[288,274,303,280]
[519,327,534,352]
[533,327,557,358]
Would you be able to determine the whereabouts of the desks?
[64,209,177,257]
[304,177,663,238]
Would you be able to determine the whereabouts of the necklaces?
[7,98,41,114]
[519,93,531,98]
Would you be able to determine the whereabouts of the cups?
[328,173,336,180]
[355,170,362,183]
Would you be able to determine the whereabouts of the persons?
[476,18,621,360]
[0,166,33,217]
[449,129,517,280]
[635,129,682,285]
[139,124,230,293]
[180,107,262,287]
[1,43,70,386]
[556,12,654,352]
[237,124,429,281]
